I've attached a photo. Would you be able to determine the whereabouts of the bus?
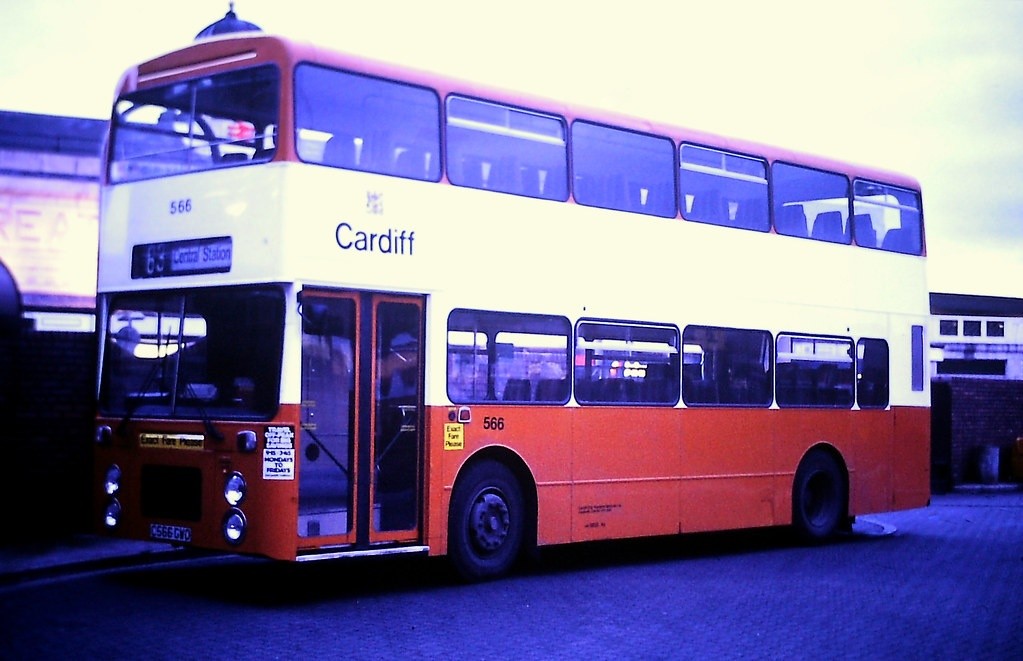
[88,29,937,582]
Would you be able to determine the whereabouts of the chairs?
[214,130,923,256]
[499,356,892,415]
